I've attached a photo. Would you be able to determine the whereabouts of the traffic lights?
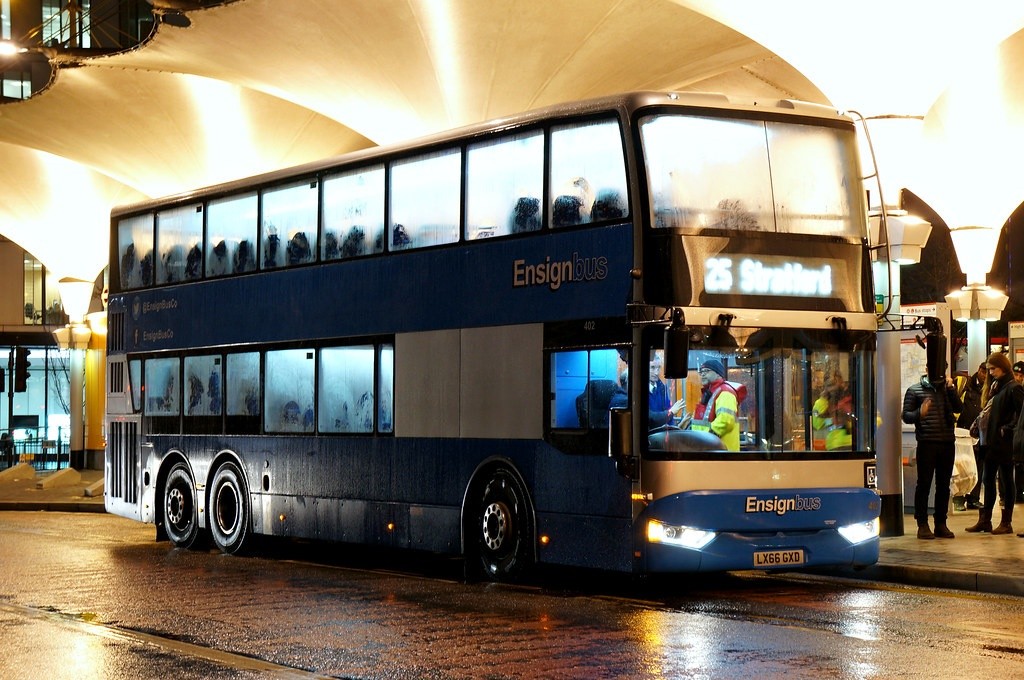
[15,347,31,392]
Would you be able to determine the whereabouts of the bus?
[102,89,947,583]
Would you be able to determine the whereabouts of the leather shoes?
[967,502,984,509]
[954,503,966,511]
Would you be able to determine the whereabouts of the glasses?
[698,369,713,376]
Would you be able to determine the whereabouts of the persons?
[812,367,882,451]
[602,353,740,452]
[956,352,1024,537]
[0,433,13,468]
[901,360,964,539]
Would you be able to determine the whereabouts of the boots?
[992,509,1013,534]
[965,508,992,532]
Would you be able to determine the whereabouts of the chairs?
[341,225,365,258]
[325,233,338,260]
[163,375,175,412]
[207,371,222,415]
[209,239,240,276]
[301,405,314,433]
[374,222,412,252]
[510,196,541,234]
[245,392,259,416]
[120,242,139,289]
[261,233,286,269]
[282,400,301,425]
[286,232,311,265]
[186,241,216,282]
[187,374,204,414]
[342,400,350,429]
[231,240,256,273]
[162,244,189,284]
[24,303,41,324]
[357,391,373,427]
[552,194,586,230]
[590,186,627,224]
[141,250,165,286]
[149,397,164,412]
[382,390,391,430]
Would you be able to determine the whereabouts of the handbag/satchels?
[969,418,980,439]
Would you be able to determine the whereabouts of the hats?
[1013,361,1024,374]
[702,360,725,377]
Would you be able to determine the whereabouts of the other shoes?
[1017,533,1024,537]
[935,525,954,538]
[917,525,935,539]
